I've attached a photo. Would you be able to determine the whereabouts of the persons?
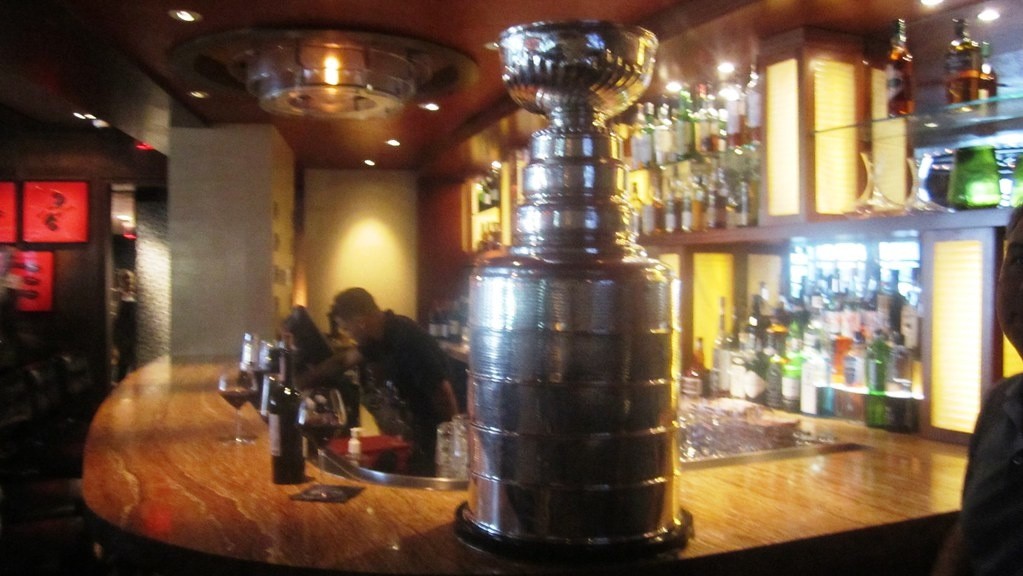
[292,287,458,443]
[932,205,1023,576]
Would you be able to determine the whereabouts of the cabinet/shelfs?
[469,0,1022,445]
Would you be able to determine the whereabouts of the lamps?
[217,39,434,118]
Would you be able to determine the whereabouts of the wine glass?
[218,370,257,444]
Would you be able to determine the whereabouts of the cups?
[241,330,260,365]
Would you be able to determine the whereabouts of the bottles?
[707,164,761,227]
[628,102,675,168]
[885,19,914,116]
[427,298,461,342]
[267,333,304,485]
[727,65,765,151]
[683,264,922,429]
[945,19,981,104]
[979,41,998,116]
[628,177,661,236]
[665,174,706,234]
[675,84,726,160]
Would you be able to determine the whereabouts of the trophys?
[453,20,693,576]
